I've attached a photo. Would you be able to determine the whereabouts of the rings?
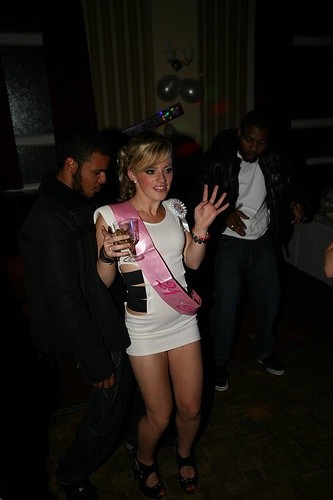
[231,224,235,228]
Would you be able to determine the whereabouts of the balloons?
[177,78,204,104]
[157,74,180,101]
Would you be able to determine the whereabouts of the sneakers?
[211,367,228,391]
[256,354,284,376]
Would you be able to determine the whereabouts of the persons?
[92,129,230,500]
[16,131,137,500]
[185,107,319,391]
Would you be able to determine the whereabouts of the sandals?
[176,454,197,494]
[132,455,164,498]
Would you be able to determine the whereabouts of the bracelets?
[191,226,210,245]
[99,245,119,265]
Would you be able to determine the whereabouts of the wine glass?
[112,216,144,262]
